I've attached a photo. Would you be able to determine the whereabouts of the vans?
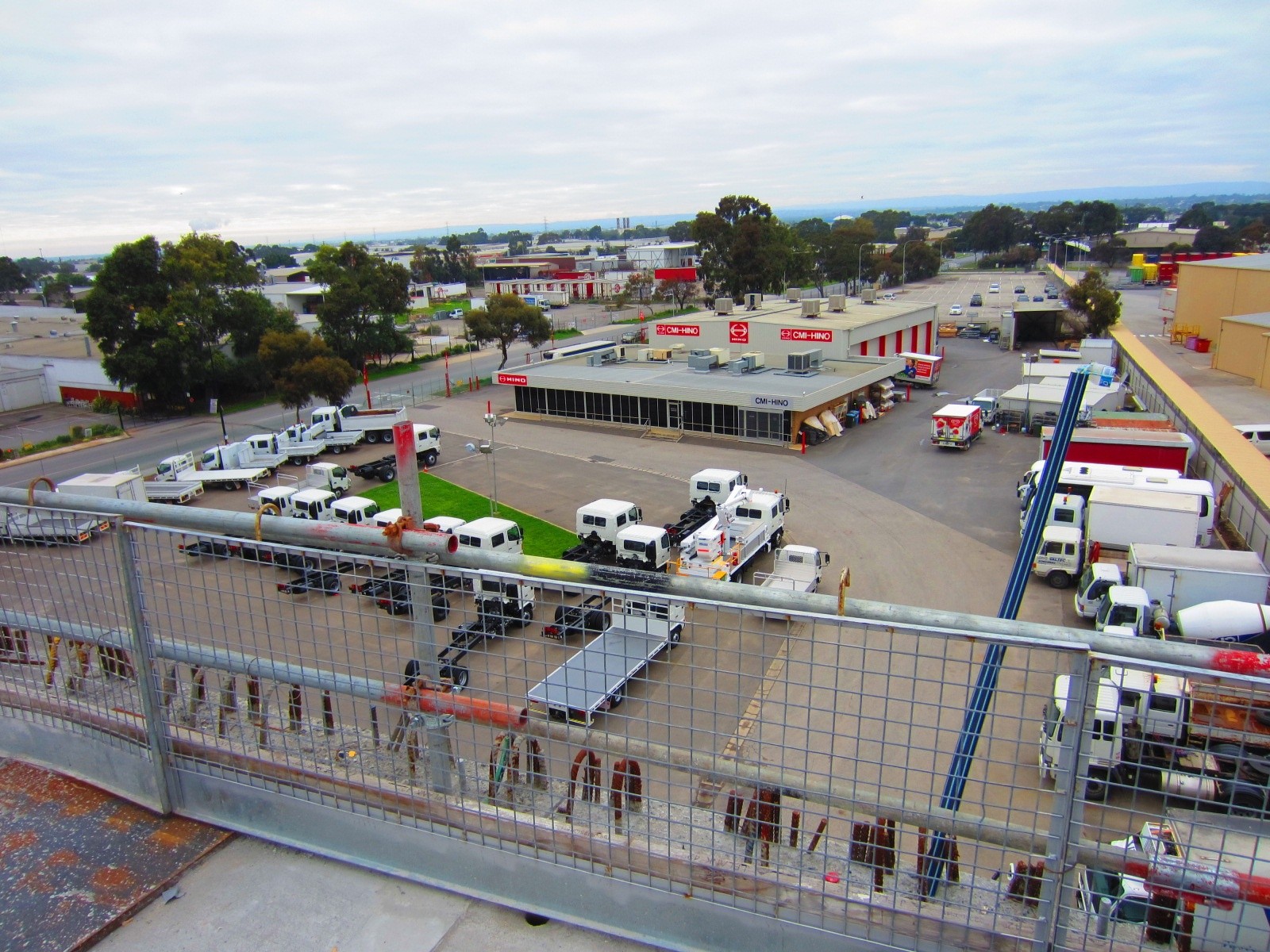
[1234,424,1270,456]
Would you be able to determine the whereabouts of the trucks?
[752,543,831,594]
[517,294,550,312]
[404,568,536,694]
[542,524,686,641]
[530,498,642,597]
[678,484,790,583]
[526,597,685,719]
[0,404,524,621]
[665,468,747,547]
[893,338,1270,952]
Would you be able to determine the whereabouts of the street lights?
[1034,231,1108,285]
[858,236,958,297]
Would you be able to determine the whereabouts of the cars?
[1048,290,1059,298]
[970,294,983,306]
[989,284,1000,292]
[1040,299,1042,299]
[1044,283,1056,292]
[1033,296,1043,301]
[1015,285,1025,293]
[883,293,897,301]
[621,330,642,343]
[1017,295,1029,302]
[949,304,963,315]
[1023,298,1025,298]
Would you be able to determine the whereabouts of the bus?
[543,341,616,360]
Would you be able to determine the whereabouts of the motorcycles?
[449,309,463,320]
